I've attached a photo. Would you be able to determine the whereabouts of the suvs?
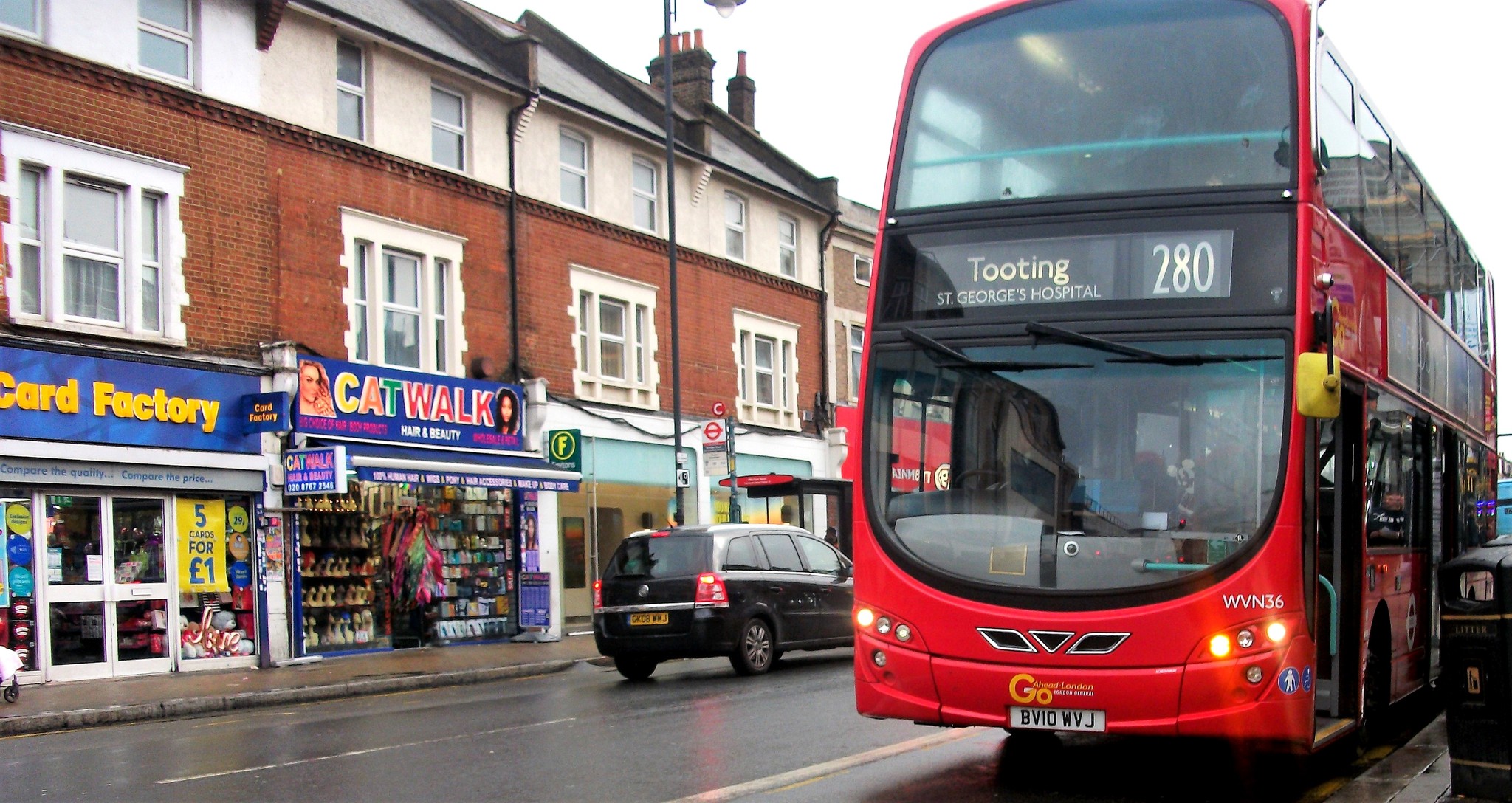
[591,523,860,678]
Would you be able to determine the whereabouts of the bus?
[1497,476,1512,538]
[815,0,1500,791]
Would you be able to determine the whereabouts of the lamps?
[346,455,357,475]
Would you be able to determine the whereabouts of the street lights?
[661,0,752,529]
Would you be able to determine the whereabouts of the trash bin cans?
[1438,533,1512,797]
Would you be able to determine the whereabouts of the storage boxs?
[149,610,166,653]
[82,614,104,641]
[435,480,512,639]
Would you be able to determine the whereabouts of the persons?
[824,527,839,548]
[495,389,518,435]
[1366,491,1411,547]
[50,522,87,584]
[525,514,539,550]
[1327,200,1439,314]
[299,361,335,417]
[234,535,244,549]
[1134,450,1179,523]
[294,480,375,648]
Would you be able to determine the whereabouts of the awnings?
[307,440,581,494]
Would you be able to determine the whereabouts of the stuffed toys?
[164,611,254,660]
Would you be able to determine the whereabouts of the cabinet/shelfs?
[432,496,511,619]
[297,481,376,650]
